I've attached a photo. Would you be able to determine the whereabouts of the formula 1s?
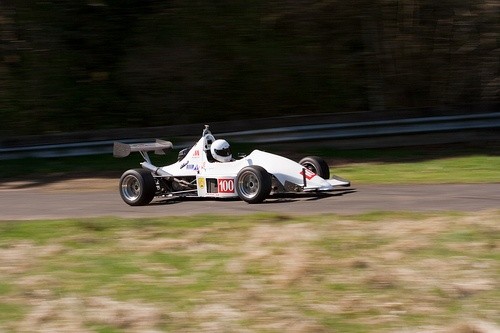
[119,125,356,207]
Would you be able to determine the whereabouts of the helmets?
[210,138,232,163]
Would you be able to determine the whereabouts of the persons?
[209,139,233,163]
